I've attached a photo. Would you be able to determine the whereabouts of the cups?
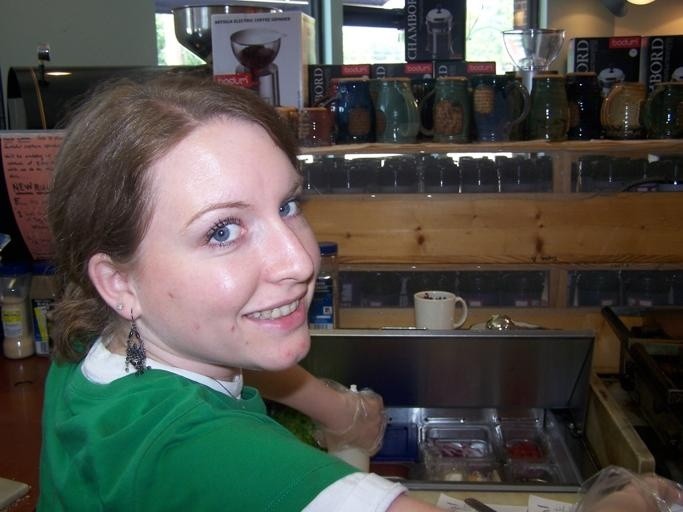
[412,291,469,332]
[275,106,337,145]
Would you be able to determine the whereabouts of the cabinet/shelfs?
[288,140,681,375]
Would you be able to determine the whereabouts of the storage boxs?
[210,9,315,108]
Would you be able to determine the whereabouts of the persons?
[33,72,683,512]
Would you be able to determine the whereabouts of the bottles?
[329,78,683,145]
[597,67,623,91]
[338,269,683,309]
[306,241,341,330]
[1,258,64,359]
[670,66,682,82]
[289,155,683,193]
[423,5,457,58]
